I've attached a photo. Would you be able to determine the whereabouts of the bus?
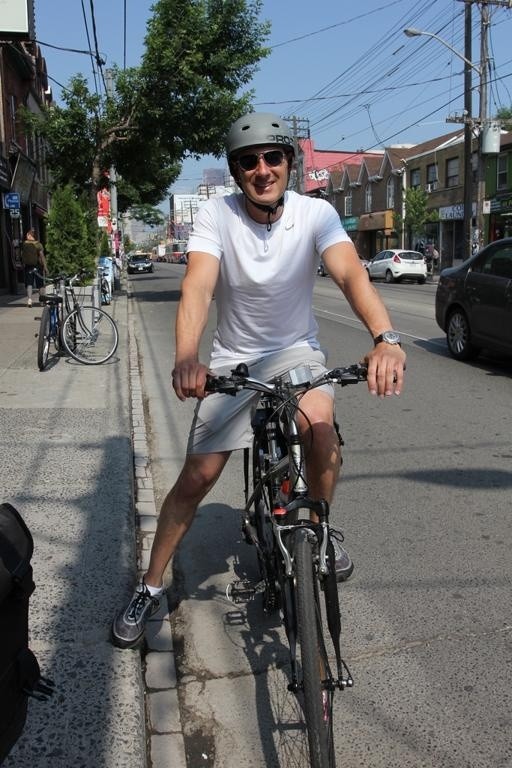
[152,239,188,263]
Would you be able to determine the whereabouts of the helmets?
[222,109,301,168]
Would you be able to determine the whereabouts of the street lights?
[403,0,492,263]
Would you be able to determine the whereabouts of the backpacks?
[22,239,41,268]
[0,502,54,768]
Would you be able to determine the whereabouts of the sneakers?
[330,534,355,584]
[27,298,34,308]
[108,576,166,651]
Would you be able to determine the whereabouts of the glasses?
[233,149,291,174]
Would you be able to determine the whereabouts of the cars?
[436,236,512,359]
[127,254,153,273]
[316,249,428,283]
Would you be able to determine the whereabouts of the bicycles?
[171,360,407,767]
[28,265,119,370]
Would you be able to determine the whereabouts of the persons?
[111,114,408,645]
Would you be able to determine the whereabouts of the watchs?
[373,329,402,346]
